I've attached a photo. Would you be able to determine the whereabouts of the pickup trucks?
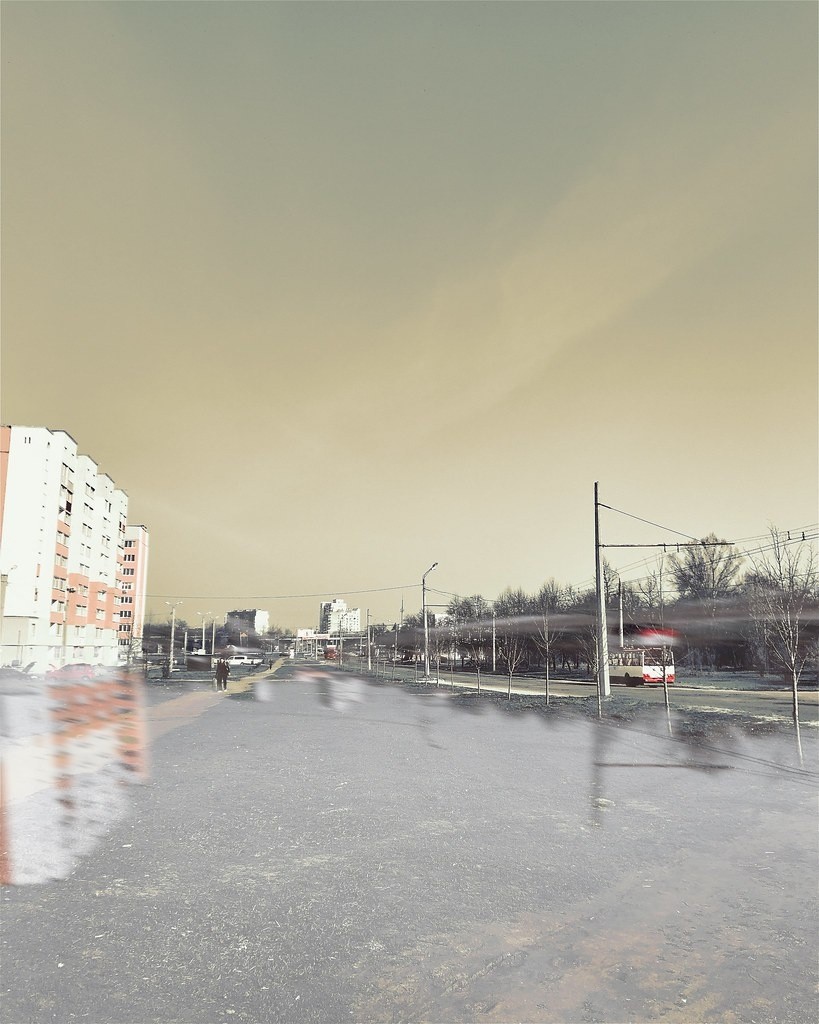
[224,656,263,665]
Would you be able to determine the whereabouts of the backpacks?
[224,661,230,676]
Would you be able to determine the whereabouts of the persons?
[269,659,273,669]
[214,658,230,693]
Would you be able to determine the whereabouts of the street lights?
[165,601,183,673]
[196,611,211,650]
[422,562,441,679]
[209,616,220,655]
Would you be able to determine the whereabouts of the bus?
[593,648,676,687]
[324,647,337,660]
